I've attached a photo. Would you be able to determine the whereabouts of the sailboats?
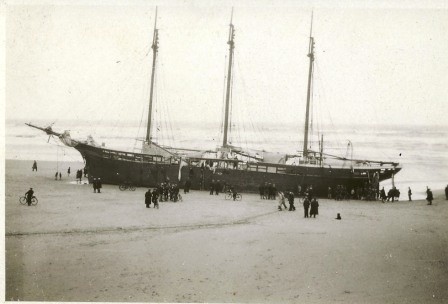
[25,3,403,201]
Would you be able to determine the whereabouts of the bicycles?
[226,189,242,201]
[154,197,159,208]
[382,195,391,202]
[19,193,38,206]
[119,180,136,191]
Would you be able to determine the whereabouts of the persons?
[93,177,97,193]
[278,191,283,211]
[381,186,385,202]
[387,187,396,202]
[351,188,355,199]
[25,188,34,206]
[307,185,313,202]
[55,172,58,180]
[124,176,132,189]
[144,189,152,208]
[79,169,82,181]
[67,167,70,176]
[201,161,204,170]
[288,190,296,211]
[33,161,37,171]
[209,178,215,195]
[209,159,213,168]
[152,189,159,208]
[59,172,62,179]
[280,191,287,209]
[97,177,102,193]
[427,189,432,205]
[205,159,209,166]
[355,186,378,201]
[76,169,80,179]
[408,187,412,201]
[221,179,225,193]
[303,198,310,218]
[445,185,448,200]
[233,156,238,169]
[310,197,319,218]
[157,181,179,202]
[184,181,191,194]
[231,187,237,201]
[259,182,277,200]
[328,186,332,199]
[215,179,221,195]
[298,184,301,198]
[84,167,88,177]
[331,184,349,201]
